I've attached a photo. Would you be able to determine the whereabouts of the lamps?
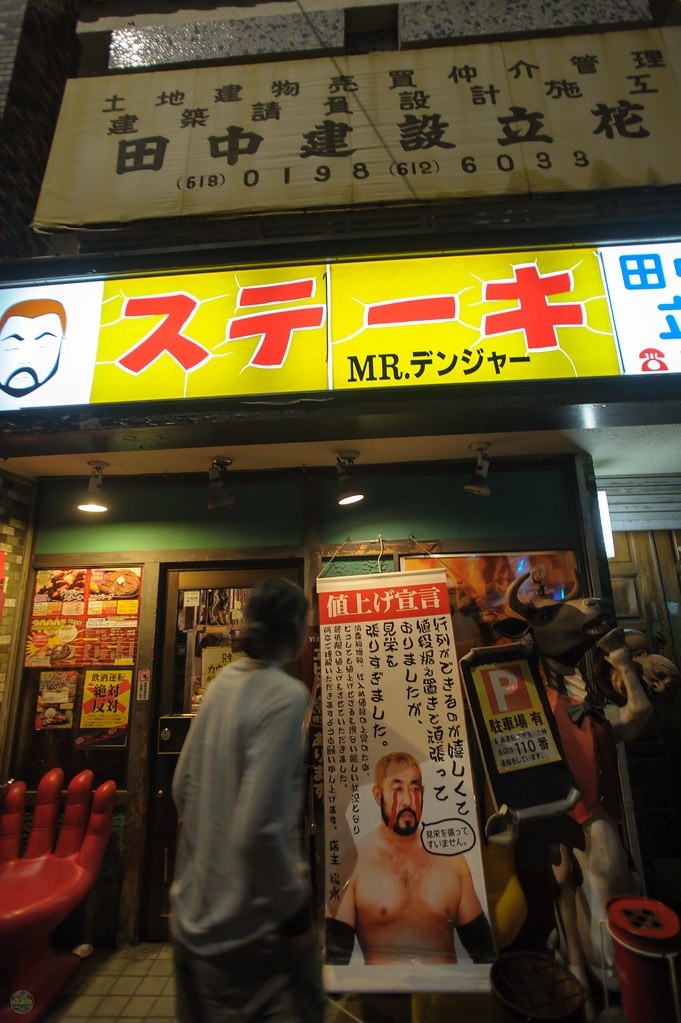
[207,457,234,511]
[76,461,109,513]
[335,449,365,507]
[599,490,617,560]
[463,441,491,496]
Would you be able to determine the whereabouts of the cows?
[491,567,655,1023]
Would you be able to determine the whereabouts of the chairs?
[0,768,118,1023]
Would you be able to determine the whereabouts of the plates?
[106,576,140,598]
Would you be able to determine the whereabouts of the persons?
[444,573,490,845]
[523,570,557,597]
[323,751,499,967]
[168,576,333,1022]
